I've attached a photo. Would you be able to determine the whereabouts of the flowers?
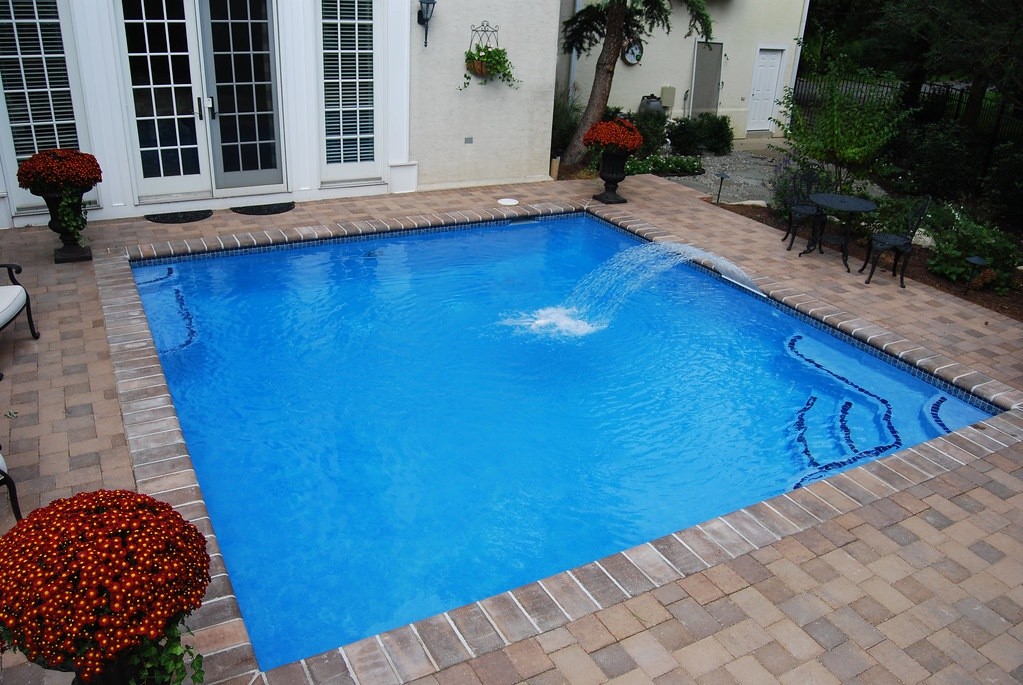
[583,118,643,154]
[16,149,103,245]
[0,489,214,684]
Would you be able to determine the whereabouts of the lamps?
[965,255,987,268]
[417,0,437,47]
[716,171,730,179]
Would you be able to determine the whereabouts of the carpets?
[230,201,295,214]
[144,209,213,223]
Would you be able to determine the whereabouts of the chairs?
[0,263,40,382]
[857,195,932,288]
[781,163,828,254]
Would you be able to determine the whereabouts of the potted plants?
[456,43,523,91]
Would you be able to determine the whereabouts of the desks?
[799,193,876,274]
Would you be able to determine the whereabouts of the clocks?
[622,39,644,65]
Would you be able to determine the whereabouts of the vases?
[594,145,630,205]
[30,181,93,263]
